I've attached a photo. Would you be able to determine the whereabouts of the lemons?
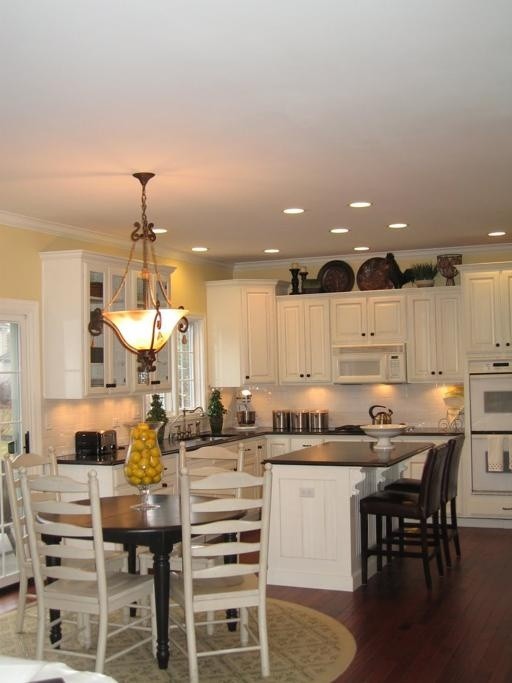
[124,423,164,485]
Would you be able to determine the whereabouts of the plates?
[317,260,355,292]
[356,257,395,291]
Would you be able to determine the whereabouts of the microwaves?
[331,345,407,384]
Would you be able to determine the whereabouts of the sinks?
[176,434,240,442]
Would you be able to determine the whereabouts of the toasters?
[74,429,118,458]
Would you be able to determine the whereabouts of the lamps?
[86,172,192,372]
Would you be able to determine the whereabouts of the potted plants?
[206,390,227,435]
[145,393,168,445]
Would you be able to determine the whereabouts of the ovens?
[469,373,512,432]
[472,435,512,492]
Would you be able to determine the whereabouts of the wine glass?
[359,424,407,449]
[437,253,463,286]
[120,420,165,510]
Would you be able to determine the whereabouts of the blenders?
[235,390,257,427]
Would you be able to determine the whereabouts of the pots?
[308,412,328,433]
[272,409,291,432]
[292,411,309,433]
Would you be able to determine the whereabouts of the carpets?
[0,594,357,683]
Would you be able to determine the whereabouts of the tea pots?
[368,403,393,424]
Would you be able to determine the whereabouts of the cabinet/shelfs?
[391,435,465,525]
[40,435,267,576]
[264,435,290,456]
[39,248,177,399]
[289,435,325,454]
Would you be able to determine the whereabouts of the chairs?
[359,434,466,592]
[19,465,158,674]
[5,446,130,649]
[137,441,244,635]
[166,461,273,682]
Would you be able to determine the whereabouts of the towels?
[487,434,504,472]
[508,435,512,470]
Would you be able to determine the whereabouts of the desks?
[260,440,434,592]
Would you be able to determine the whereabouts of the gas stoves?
[335,425,367,435]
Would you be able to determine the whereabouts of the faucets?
[183,407,204,433]
